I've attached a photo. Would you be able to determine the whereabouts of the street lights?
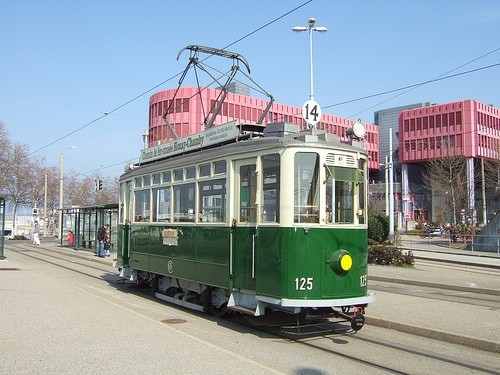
[292,18,328,130]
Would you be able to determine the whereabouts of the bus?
[114,119,376,336]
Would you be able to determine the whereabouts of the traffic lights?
[99,180,103,191]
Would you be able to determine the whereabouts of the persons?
[98,224,111,257]
[67,231,73,244]
[33,221,40,245]
